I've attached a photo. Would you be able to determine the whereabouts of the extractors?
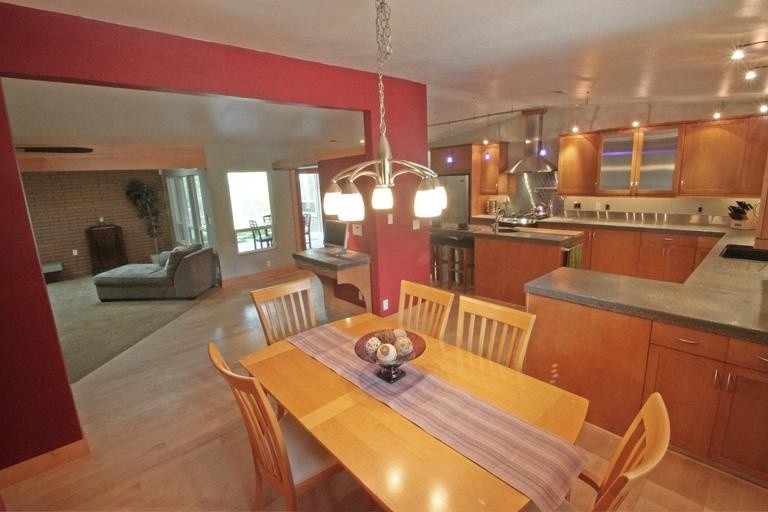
[503,114,558,175]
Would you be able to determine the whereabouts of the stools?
[429,236,474,294]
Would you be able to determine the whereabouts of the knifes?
[727,200,752,220]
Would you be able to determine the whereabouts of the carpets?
[43,274,220,383]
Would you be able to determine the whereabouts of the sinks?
[494,225,522,233]
[719,243,768,263]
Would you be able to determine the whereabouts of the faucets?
[494,210,507,235]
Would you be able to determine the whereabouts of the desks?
[292,246,372,315]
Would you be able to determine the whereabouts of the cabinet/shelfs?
[538,223,721,284]
[480,141,507,195]
[557,131,596,195]
[637,321,767,492]
[596,113,767,197]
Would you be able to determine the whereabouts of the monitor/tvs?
[323,220,348,250]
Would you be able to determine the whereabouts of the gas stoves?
[502,212,549,221]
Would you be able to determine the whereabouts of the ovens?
[496,218,538,228]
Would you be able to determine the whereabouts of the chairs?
[94,243,215,301]
[248,214,311,251]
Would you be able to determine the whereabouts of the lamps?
[321,0,448,225]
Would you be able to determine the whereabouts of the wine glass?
[354,328,427,383]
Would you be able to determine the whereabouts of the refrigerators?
[429,175,471,286]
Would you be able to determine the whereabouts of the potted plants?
[124,179,165,263]
